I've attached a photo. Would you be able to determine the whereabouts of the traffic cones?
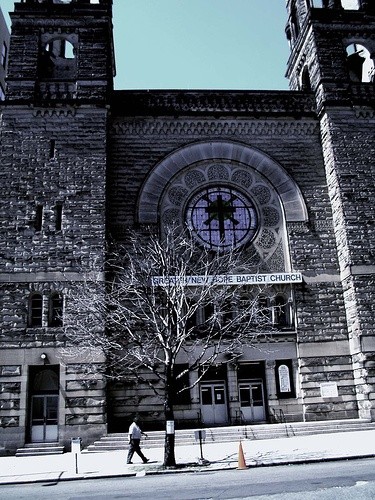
[236,442,249,470]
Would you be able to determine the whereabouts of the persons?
[127,417,150,464]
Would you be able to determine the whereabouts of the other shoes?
[142,458,149,463]
[127,461,133,464]
